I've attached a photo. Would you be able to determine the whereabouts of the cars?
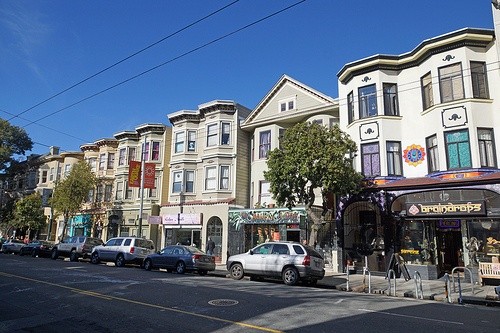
[51,236,103,261]
[143,245,215,275]
[2,240,54,257]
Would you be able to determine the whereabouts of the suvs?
[227,241,325,285]
[91,237,155,267]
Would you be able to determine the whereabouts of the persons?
[206,236,215,255]
[0,233,38,244]
